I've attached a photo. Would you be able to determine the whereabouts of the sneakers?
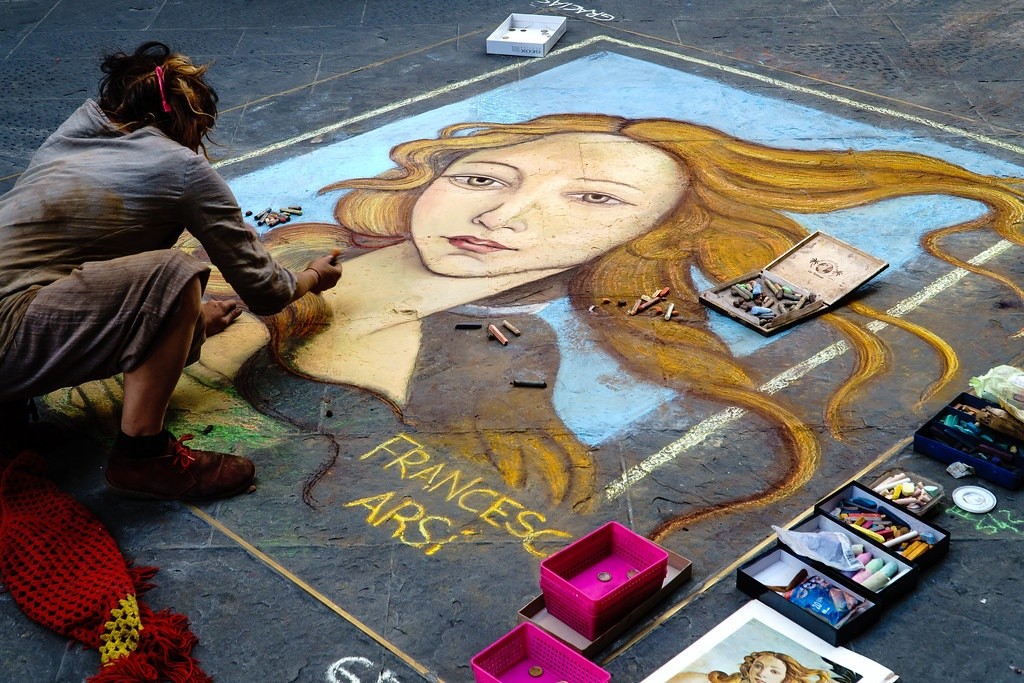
[105,428,256,503]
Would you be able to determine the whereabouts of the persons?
[0,41,343,502]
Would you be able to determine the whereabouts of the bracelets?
[302,267,321,284]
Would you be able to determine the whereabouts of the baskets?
[539,521,669,643]
[470,622,611,683]
